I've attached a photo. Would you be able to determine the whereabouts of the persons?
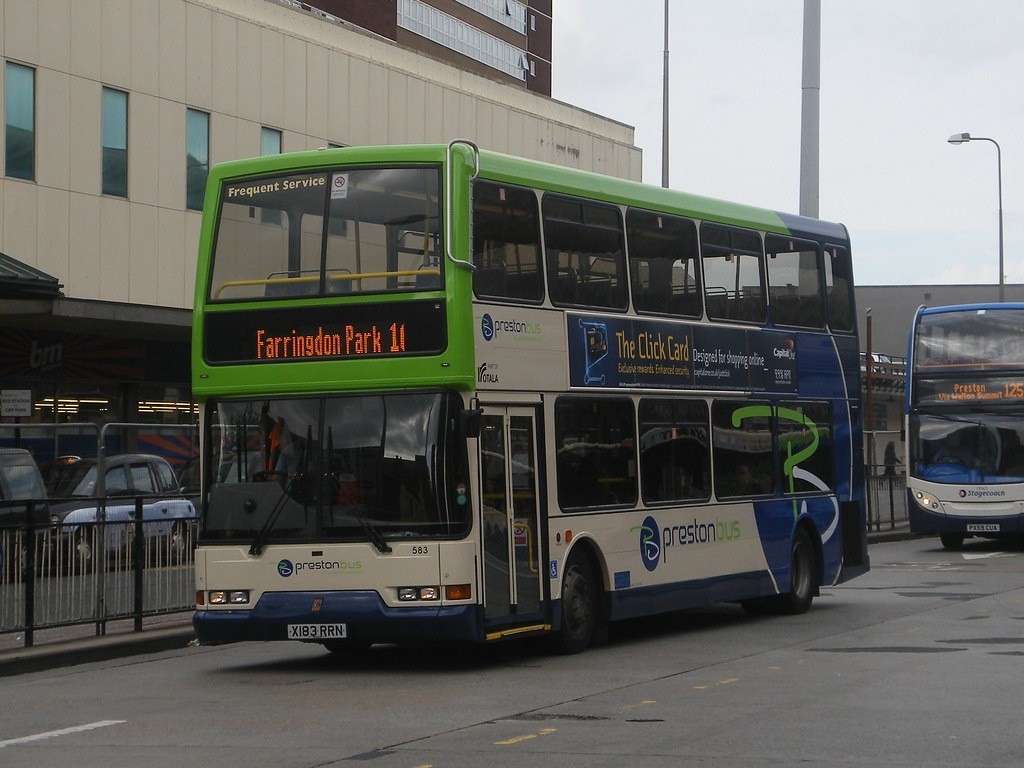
[273,422,337,505]
[877,439,905,486]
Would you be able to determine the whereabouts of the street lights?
[943,134,1007,304]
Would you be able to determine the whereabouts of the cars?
[44,456,196,573]
[2,446,54,581]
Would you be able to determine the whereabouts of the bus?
[905,297,1024,552]
[183,135,871,651]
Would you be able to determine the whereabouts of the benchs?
[264,269,837,336]
[926,357,991,365]
